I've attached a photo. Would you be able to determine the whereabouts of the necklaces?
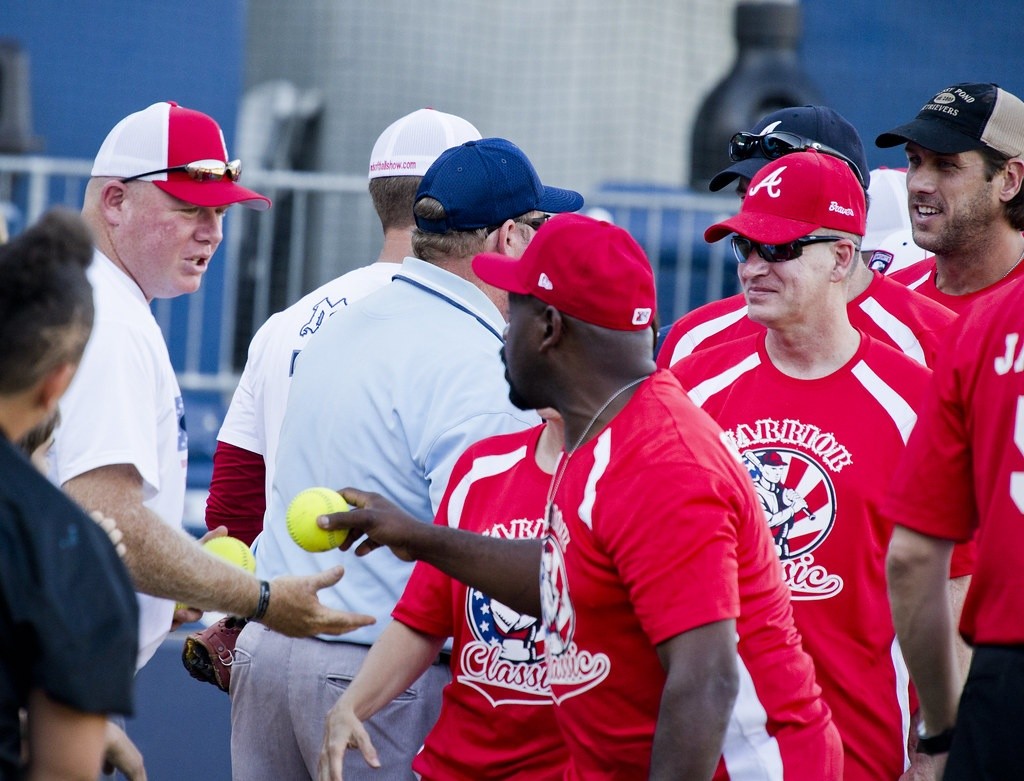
[542,375,656,546]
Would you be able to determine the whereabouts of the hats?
[90,100,272,213]
[875,82,1024,161]
[369,106,482,180]
[709,104,870,192]
[704,147,866,245]
[471,213,656,331]
[413,138,584,234]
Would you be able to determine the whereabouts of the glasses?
[728,131,865,188]
[120,159,242,183]
[731,236,860,263]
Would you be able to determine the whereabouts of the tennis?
[202,535,257,582]
[287,486,349,552]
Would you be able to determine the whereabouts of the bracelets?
[245,580,270,622]
[916,728,954,756]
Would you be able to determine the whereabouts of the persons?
[0,81,1024,781]
